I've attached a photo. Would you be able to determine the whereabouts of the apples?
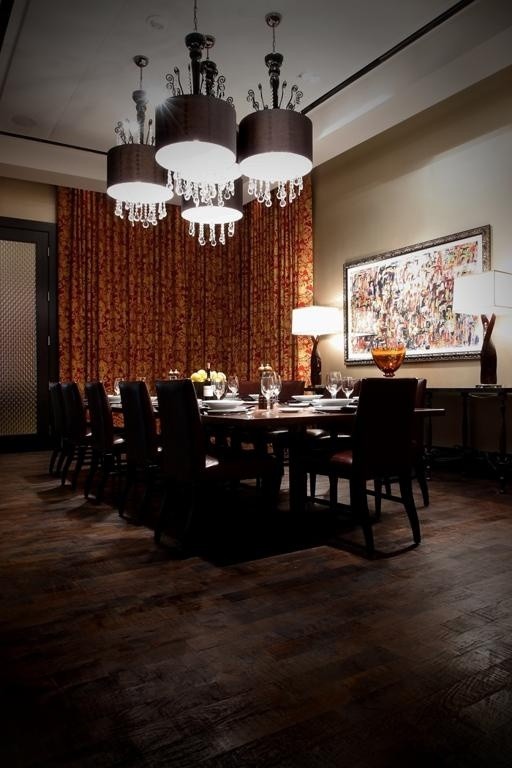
[190,369,226,383]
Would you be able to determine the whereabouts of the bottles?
[203,360,213,400]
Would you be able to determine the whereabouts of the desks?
[424,387,509,494]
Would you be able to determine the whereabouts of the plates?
[312,396,354,407]
[149,396,157,400]
[104,394,122,403]
[247,392,259,401]
[292,394,324,400]
[203,398,245,409]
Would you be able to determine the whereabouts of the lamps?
[451,266,511,387]
[290,304,341,385]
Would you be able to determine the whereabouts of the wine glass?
[138,375,147,383]
[323,369,355,398]
[259,367,282,411]
[114,376,126,397]
[213,373,239,398]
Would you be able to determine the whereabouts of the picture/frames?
[342,225,491,366]
